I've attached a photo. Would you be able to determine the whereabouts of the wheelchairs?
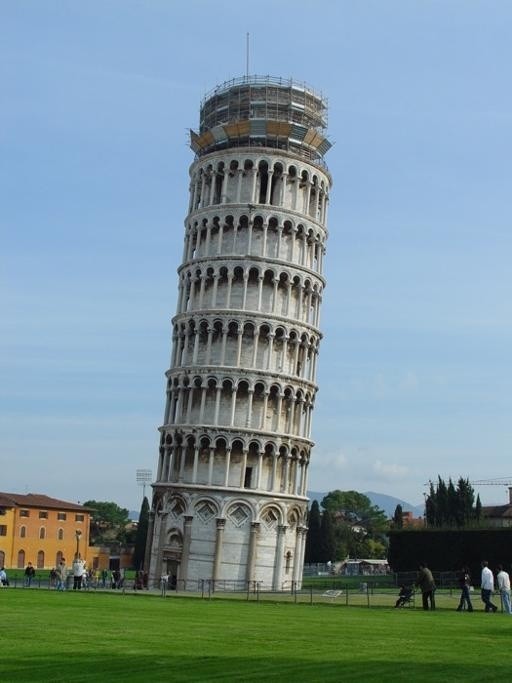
[394,583,416,608]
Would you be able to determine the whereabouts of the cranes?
[423,477,512,486]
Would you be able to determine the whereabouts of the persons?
[415,562,437,610]
[49,557,171,592]
[24,562,35,588]
[456,567,473,611]
[394,585,407,608]
[496,565,512,613]
[480,561,498,613]
[1,567,6,587]
